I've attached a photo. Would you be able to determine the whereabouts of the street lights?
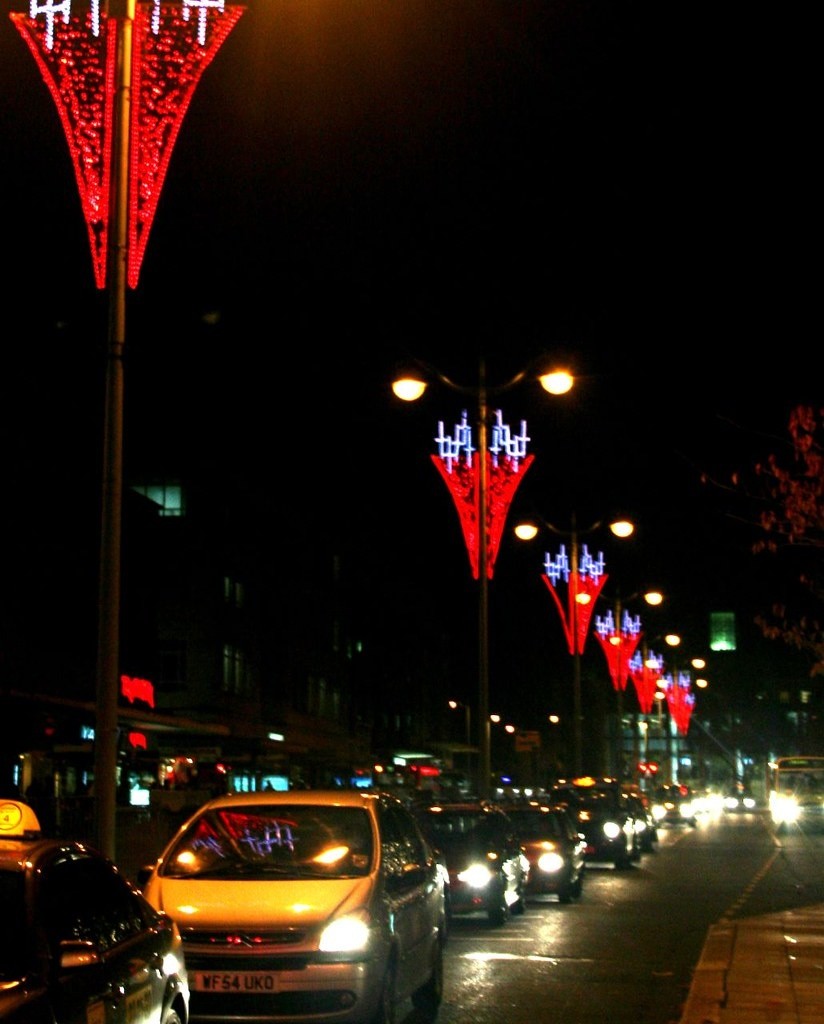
[388,347,576,801]
[512,496,635,781]
[3,0,244,853]
[575,574,667,781]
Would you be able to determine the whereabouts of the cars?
[405,798,531,926]
[1,800,192,1024]
[139,789,451,1024]
[466,756,824,917]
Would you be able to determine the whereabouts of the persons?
[74,776,87,798]
[149,778,171,791]
[24,777,45,824]
[174,774,210,791]
